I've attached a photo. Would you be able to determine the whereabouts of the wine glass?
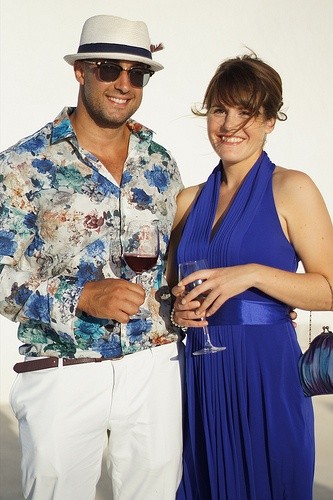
[123,221,160,319]
[178,258,226,355]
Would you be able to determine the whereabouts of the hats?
[63,15,164,71]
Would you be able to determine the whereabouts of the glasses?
[83,60,155,87]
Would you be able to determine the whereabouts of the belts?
[13,355,124,373]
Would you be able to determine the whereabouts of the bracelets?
[170,305,188,332]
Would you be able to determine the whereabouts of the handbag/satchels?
[297,273,333,398]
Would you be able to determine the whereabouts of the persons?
[0,13,297,500]
[164,56,333,500]
